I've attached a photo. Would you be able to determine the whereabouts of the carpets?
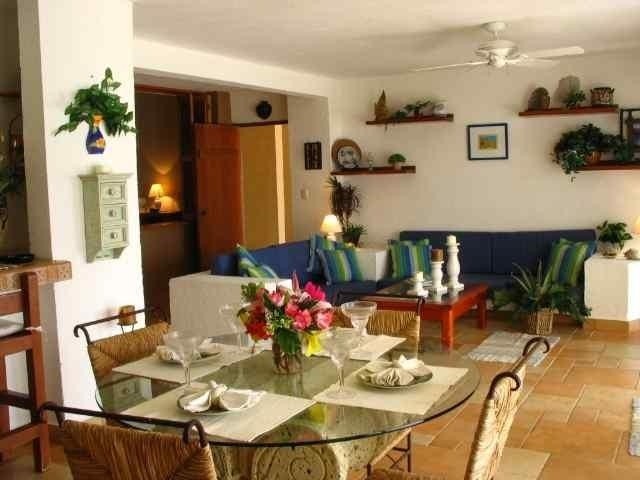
[628,397,640,457]
[467,329,561,366]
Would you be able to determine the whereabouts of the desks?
[94,334,482,480]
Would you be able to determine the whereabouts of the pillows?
[388,229,597,288]
[211,232,367,287]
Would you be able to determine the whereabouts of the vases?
[272,339,307,374]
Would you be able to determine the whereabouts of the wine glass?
[161,331,207,390]
[340,299,381,349]
[218,302,253,357]
[317,328,365,402]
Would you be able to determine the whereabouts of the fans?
[410,22,585,73]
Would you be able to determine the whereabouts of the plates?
[154,351,221,364]
[558,75,580,103]
[174,393,231,417]
[351,366,436,392]
[331,139,364,170]
[527,87,548,109]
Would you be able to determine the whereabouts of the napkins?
[155,341,220,362]
[181,378,260,413]
[364,353,428,388]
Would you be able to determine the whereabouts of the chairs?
[73,304,241,479]
[360,336,550,480]
[0,273,50,472]
[36,399,243,480]
[313,293,423,476]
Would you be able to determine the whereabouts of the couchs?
[377,230,597,314]
[169,239,377,340]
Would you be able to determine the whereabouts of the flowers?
[238,282,336,355]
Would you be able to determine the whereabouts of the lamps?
[320,215,344,242]
[147,184,165,210]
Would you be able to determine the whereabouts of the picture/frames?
[305,143,322,169]
[467,123,508,160]
[620,108,640,163]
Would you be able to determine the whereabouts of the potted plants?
[385,96,444,132]
[597,220,633,259]
[55,67,143,155]
[485,256,592,334]
[325,176,368,247]
[562,91,586,109]
[553,123,634,182]
[388,154,407,171]
[527,88,551,109]
